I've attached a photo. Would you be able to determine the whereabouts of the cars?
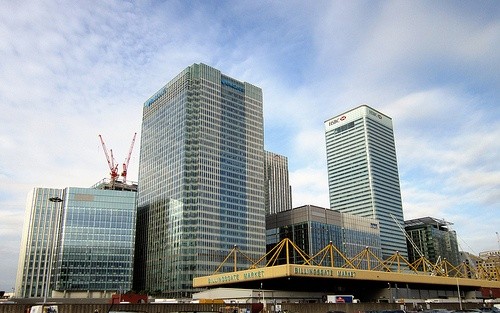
[327,306,500,313]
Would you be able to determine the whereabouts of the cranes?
[99,133,137,191]
